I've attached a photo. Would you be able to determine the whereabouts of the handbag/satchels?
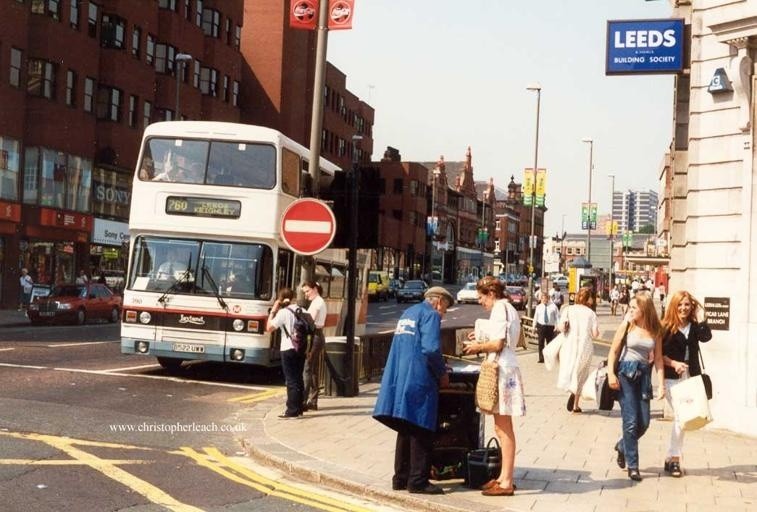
[564,319,569,332]
[465,447,502,489]
[473,360,499,412]
[670,374,713,431]
[595,361,615,410]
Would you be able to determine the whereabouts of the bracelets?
[270,311,278,314]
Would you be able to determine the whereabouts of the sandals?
[665,459,682,477]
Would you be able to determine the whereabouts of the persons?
[661,291,712,477]
[532,275,665,412]
[372,286,455,493]
[155,247,195,285]
[461,276,526,496]
[301,281,326,412]
[267,288,308,421]
[608,290,666,480]
[18,262,106,318]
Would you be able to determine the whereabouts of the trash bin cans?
[525,304,536,319]
[323,336,360,397]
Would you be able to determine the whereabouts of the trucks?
[366,269,390,302]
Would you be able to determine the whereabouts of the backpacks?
[285,308,315,354]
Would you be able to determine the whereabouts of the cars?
[395,280,429,303]
[27,281,121,324]
[387,279,401,294]
[459,270,569,309]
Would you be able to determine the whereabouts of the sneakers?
[409,483,441,494]
[567,393,582,412]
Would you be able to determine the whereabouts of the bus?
[117,119,376,379]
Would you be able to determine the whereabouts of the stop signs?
[277,196,337,256]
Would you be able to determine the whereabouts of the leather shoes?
[482,479,514,495]
[279,402,317,417]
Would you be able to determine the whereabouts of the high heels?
[628,467,642,480]
[615,444,625,468]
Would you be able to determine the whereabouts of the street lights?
[581,139,594,262]
[425,166,442,284]
[478,190,492,273]
[523,83,543,305]
[559,213,569,255]
[606,173,615,300]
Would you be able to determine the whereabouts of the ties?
[544,304,548,322]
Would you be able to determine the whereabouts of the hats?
[424,286,454,308]
